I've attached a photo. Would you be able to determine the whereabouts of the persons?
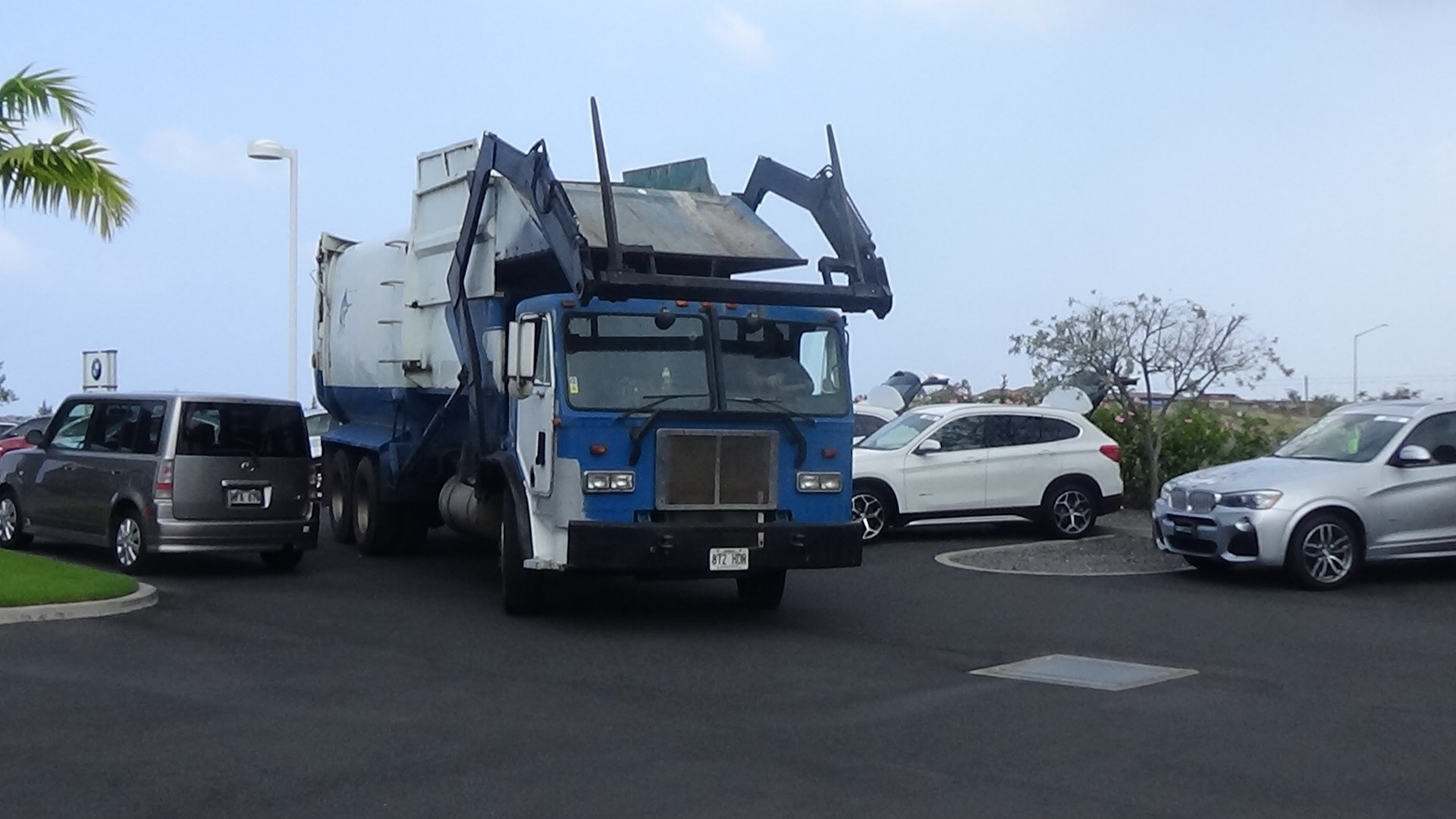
[1384,425,1438,464]
[743,329,814,395]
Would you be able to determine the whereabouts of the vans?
[1,390,317,576]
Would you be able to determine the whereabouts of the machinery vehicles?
[310,98,898,615]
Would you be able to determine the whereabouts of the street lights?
[246,138,300,400]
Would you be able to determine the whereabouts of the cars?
[852,371,946,448]
[0,416,85,458]
[23,414,222,450]
[303,408,344,492]
[849,369,1140,545]
[1150,398,1455,592]
